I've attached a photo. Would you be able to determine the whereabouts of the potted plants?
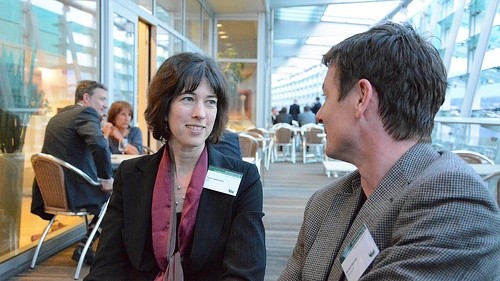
[0,44,47,257]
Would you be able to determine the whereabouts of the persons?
[101,101,143,155]
[276,20,500,281]
[30,80,114,267]
[272,97,323,153]
[210,114,242,161]
[83,52,266,281]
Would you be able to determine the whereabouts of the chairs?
[237,123,327,182]
[30,144,155,280]
[452,150,500,211]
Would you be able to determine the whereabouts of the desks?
[324,160,358,178]
[468,164,500,202]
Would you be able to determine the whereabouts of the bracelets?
[121,139,127,147]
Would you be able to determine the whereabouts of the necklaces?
[175,174,186,205]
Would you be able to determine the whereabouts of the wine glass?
[117,139,128,156]
